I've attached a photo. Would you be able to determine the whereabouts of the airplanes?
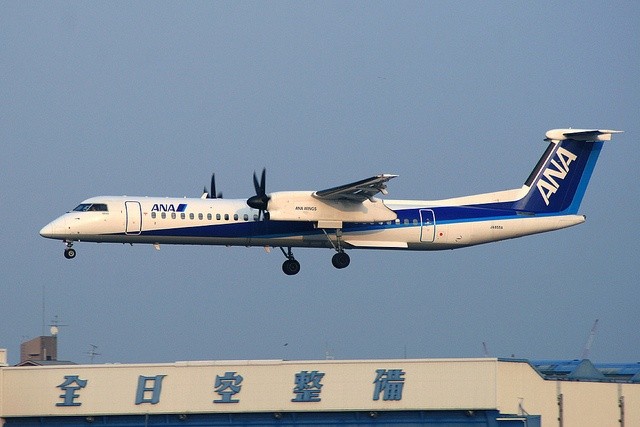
[38,126,626,275]
